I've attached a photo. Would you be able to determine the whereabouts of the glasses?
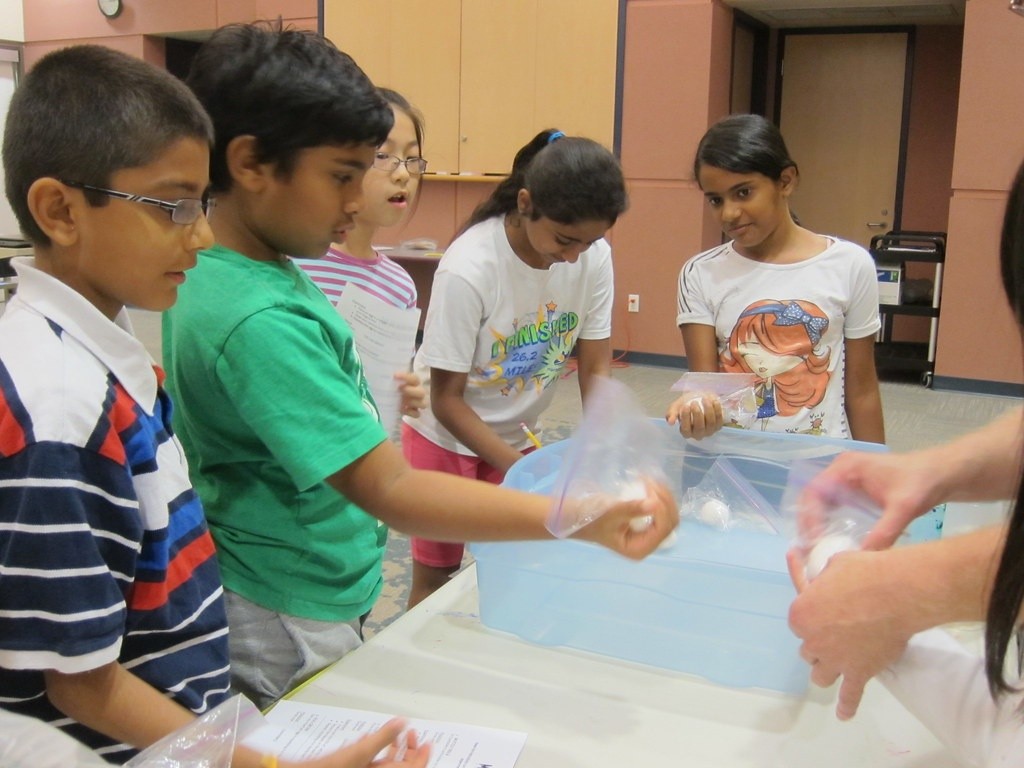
[55,179,220,226]
[376,152,429,176]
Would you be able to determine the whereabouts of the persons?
[787,161,1024,768]
[160,23,681,710]
[280,87,427,446]
[0,44,435,768]
[401,128,630,616]
[663,114,886,448]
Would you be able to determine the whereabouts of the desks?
[178,494,1024,768]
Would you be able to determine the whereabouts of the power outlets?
[628,295,640,313]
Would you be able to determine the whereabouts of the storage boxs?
[466,417,893,702]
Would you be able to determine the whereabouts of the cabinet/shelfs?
[869,230,947,387]
